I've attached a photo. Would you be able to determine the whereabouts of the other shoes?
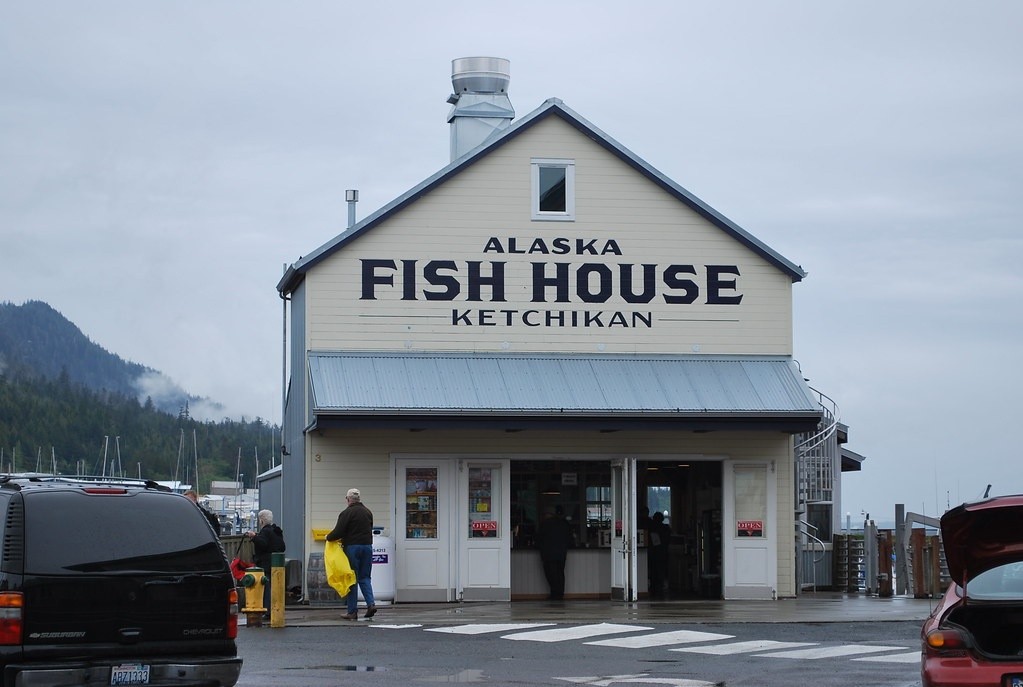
[341,612,358,619]
[544,595,565,601]
[364,604,377,617]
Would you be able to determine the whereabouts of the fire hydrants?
[240,564,268,629]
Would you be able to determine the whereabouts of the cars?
[919,490,1023,687]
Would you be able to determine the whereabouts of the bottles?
[408,528,436,538]
[407,480,436,492]
[469,498,490,512]
[472,489,491,496]
[409,512,430,524]
[408,496,434,510]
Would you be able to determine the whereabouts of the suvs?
[0,474,243,687]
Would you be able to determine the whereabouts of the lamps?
[281,445,292,456]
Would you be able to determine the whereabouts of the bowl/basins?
[410,524,430,527]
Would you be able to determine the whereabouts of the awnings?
[305,351,825,437]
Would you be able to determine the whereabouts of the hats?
[346,489,360,498]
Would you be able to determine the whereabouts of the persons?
[639,505,672,548]
[244,509,286,622]
[537,511,569,604]
[324,488,378,620]
[183,489,220,538]
